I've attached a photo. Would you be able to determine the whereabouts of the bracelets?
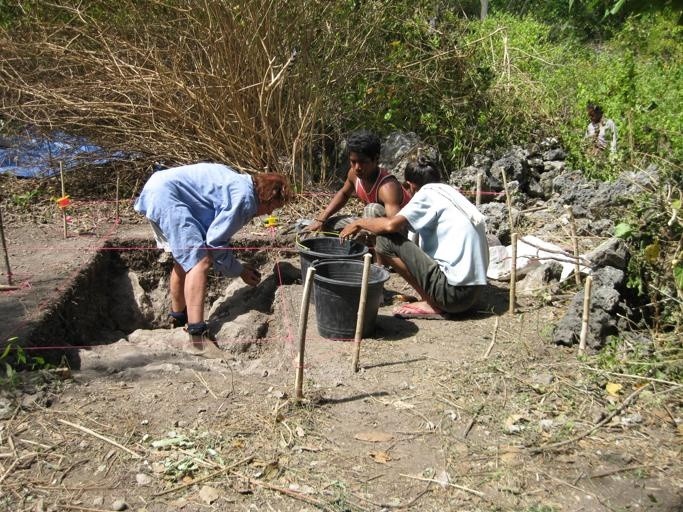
[314,219,324,223]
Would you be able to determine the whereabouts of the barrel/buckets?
[310,258,390,340]
[295,229,369,304]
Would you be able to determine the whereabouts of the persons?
[132,162,292,349]
[586,103,617,173]
[297,128,413,308]
[338,157,491,321]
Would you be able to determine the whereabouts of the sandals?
[391,303,447,321]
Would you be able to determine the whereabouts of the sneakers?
[182,327,234,361]
